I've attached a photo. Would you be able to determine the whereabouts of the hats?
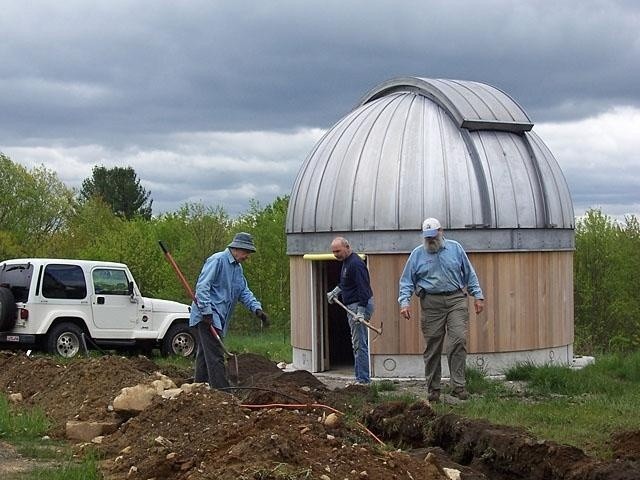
[227,232,257,253]
[422,218,442,238]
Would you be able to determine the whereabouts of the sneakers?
[429,391,440,401]
[450,387,472,400]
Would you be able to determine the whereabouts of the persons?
[396,218,486,404]
[188,230,270,395]
[325,235,377,390]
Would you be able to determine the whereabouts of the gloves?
[203,314,213,329]
[255,309,269,326]
[352,306,365,325]
[327,286,342,304]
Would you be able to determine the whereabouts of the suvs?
[0,258,196,361]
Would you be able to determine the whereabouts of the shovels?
[159,239,239,380]
